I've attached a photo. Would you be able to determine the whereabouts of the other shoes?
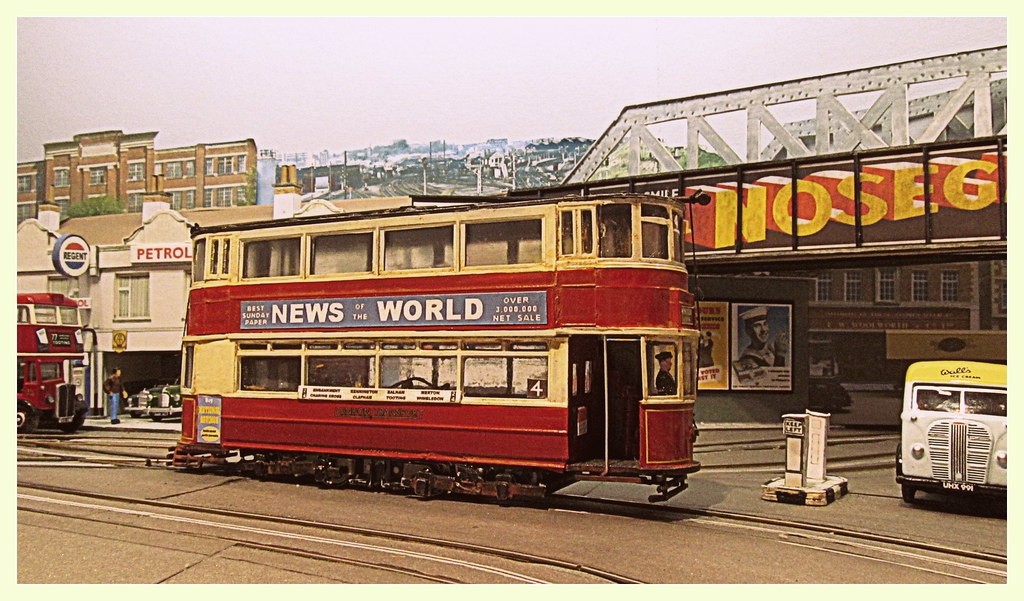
[111,419,119,424]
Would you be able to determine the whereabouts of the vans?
[893,360,1007,506]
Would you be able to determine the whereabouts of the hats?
[739,307,767,322]
[655,352,673,361]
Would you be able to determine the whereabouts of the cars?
[127,373,184,421]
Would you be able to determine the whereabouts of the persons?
[739,306,788,372]
[103,367,128,424]
[655,352,688,394]
[314,360,364,388]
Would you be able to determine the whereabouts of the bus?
[17,292,100,433]
[159,187,705,502]
